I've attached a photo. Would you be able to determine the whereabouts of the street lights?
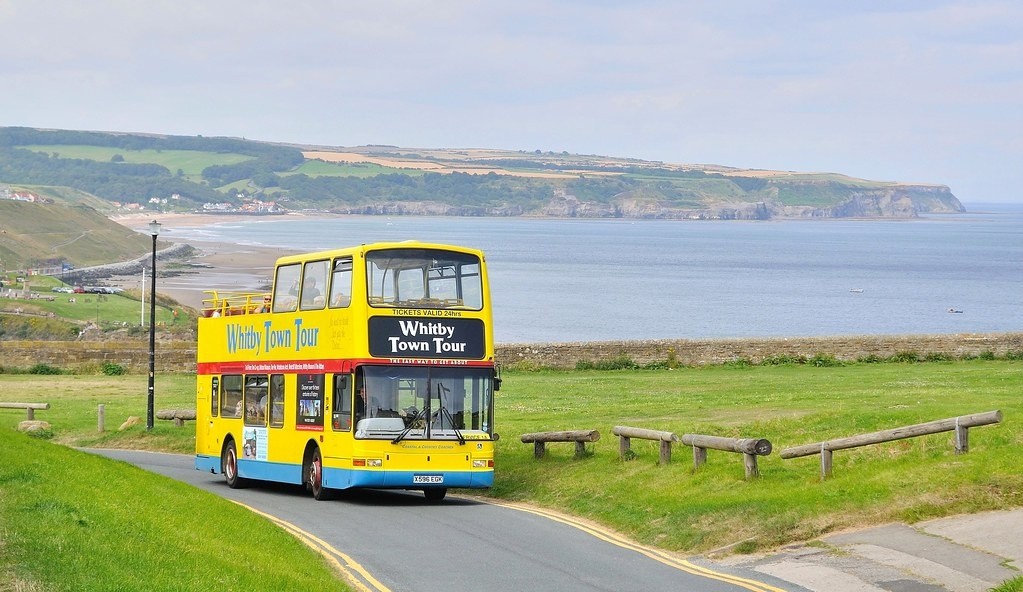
[147,220,162,430]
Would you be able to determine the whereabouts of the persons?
[357,385,378,411]
[283,276,344,313]
[254,294,272,314]
[212,302,229,317]
[260,394,281,422]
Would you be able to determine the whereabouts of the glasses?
[264,298,272,301]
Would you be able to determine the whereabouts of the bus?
[195,241,502,501]
